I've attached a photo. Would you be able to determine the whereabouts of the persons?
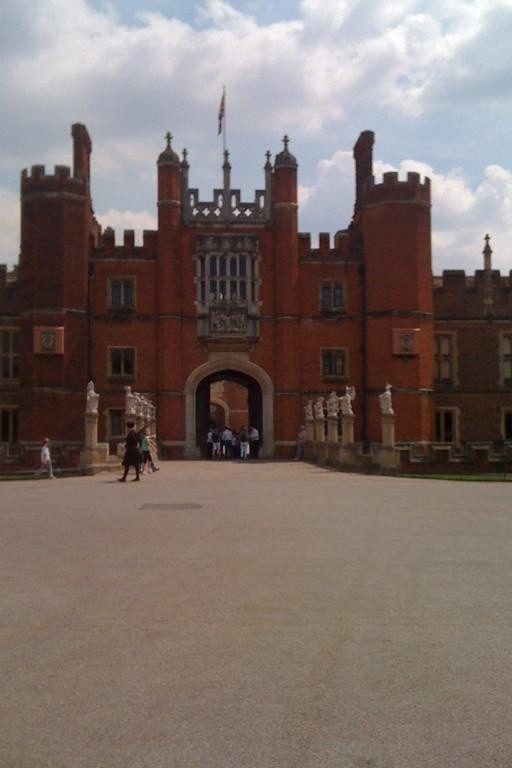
[34,437,58,479]
[206,425,259,460]
[117,420,160,481]
[292,426,306,461]
